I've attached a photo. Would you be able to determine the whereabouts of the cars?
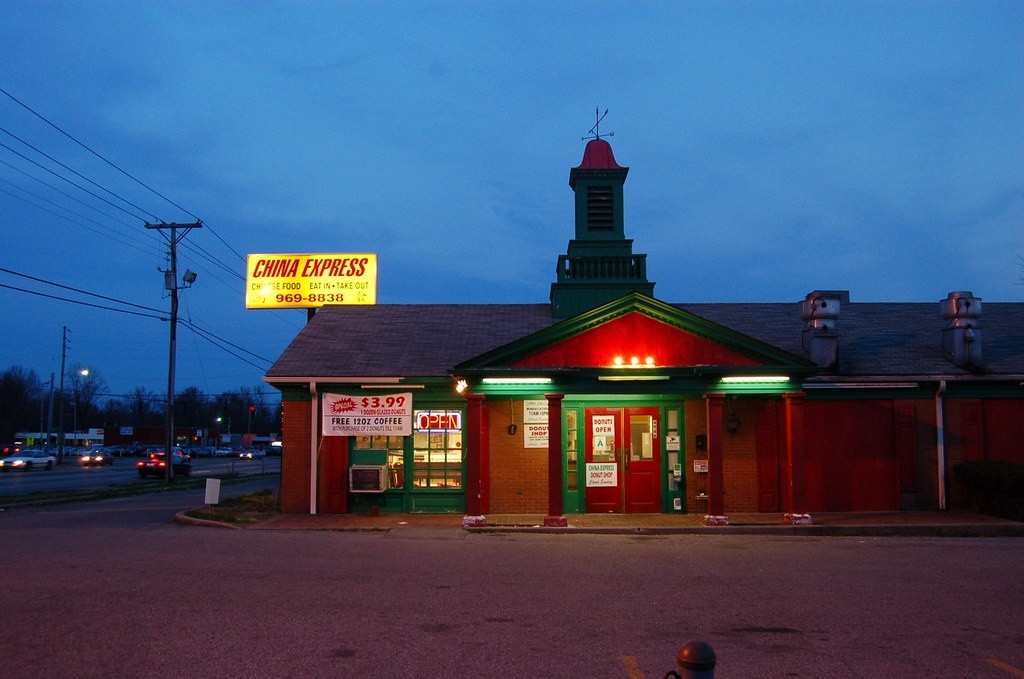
[137,452,192,479]
[0,442,281,460]
[82,451,114,467]
[0,450,57,473]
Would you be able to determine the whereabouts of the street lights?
[165,269,198,485]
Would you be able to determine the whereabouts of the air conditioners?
[349,465,387,493]
